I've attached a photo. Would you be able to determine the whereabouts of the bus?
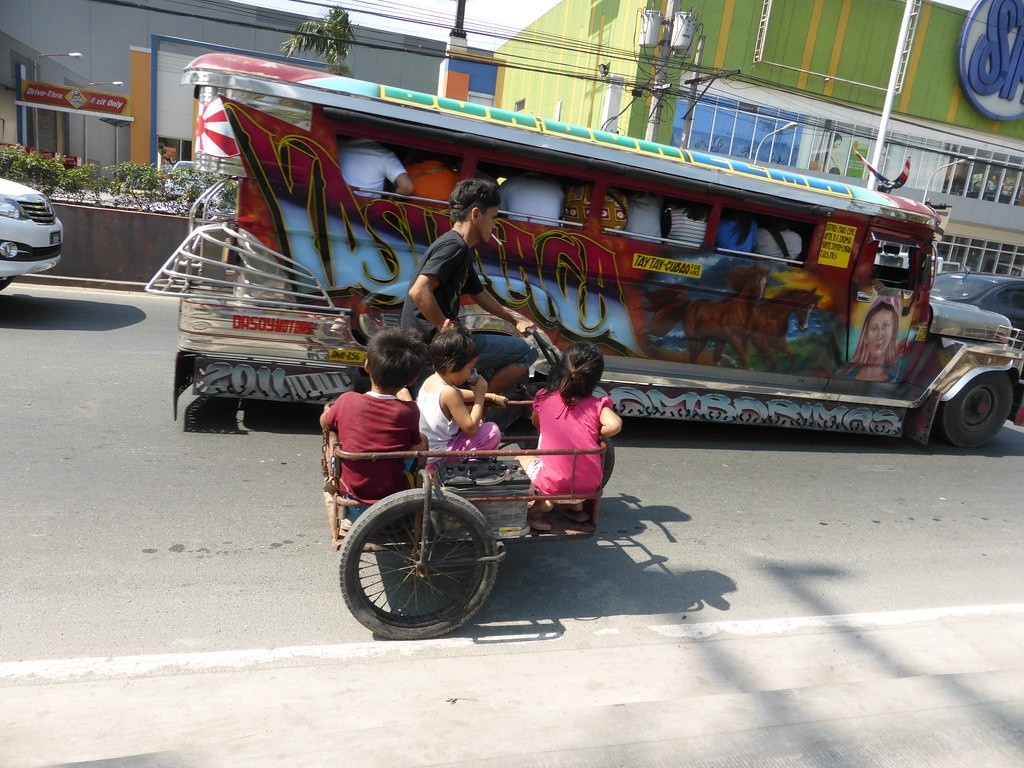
[145,51,1024,457]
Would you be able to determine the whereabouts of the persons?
[335,136,805,266]
[418,330,510,486]
[163,157,173,175]
[399,177,539,409]
[318,328,428,511]
[514,340,625,531]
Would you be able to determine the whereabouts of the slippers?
[555,506,589,521]
[528,515,551,531]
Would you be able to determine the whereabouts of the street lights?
[923,156,969,205]
[753,120,800,164]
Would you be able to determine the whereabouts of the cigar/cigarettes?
[492,233,504,245]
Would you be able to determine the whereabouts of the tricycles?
[321,320,615,640]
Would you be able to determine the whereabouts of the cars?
[165,161,198,197]
[34,53,83,158]
[0,177,64,291]
[930,271,1024,348]
